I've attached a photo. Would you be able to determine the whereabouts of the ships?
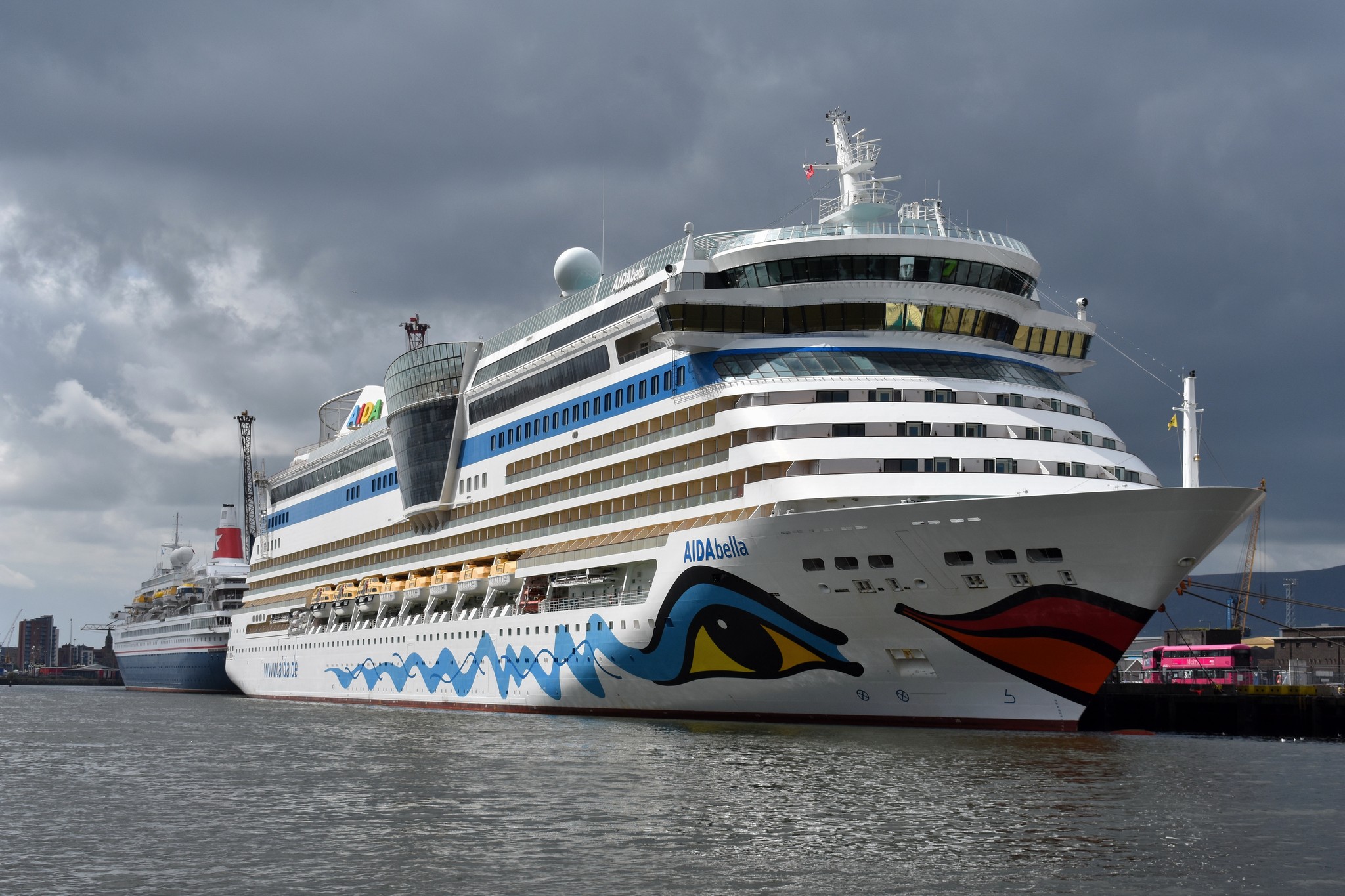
[225,104,1270,737]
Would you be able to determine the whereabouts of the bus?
[1158,644,1254,686]
[1140,645,1168,684]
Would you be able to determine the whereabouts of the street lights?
[73,638,77,665]
[43,651,47,677]
[68,618,74,667]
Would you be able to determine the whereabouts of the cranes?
[1231,473,1266,634]
[4,626,15,664]
[0,609,23,652]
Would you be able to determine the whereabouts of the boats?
[488,559,529,594]
[355,577,385,615]
[310,585,335,619]
[403,575,442,605]
[331,581,358,618]
[174,583,205,604]
[152,591,165,606]
[457,564,499,599]
[132,594,154,609]
[162,586,180,605]
[429,570,470,603]
[378,578,415,609]
[109,502,250,697]
[519,587,558,614]
